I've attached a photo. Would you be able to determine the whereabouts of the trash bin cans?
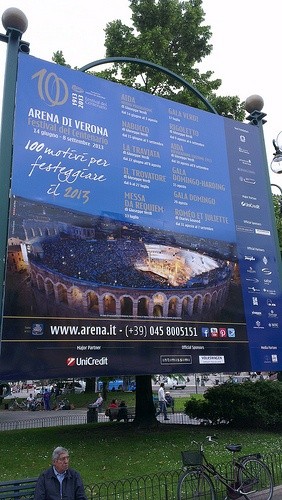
[86,404,99,423]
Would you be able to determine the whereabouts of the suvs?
[34,386,56,397]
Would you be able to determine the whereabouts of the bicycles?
[177,434,273,500]
[8,396,28,411]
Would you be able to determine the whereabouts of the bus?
[97,377,136,392]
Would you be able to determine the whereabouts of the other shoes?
[164,418,170,420]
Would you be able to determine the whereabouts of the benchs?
[0,478,41,500]
[152,400,174,414]
[109,407,135,423]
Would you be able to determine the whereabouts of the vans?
[231,376,250,385]
[55,381,85,395]
[160,376,186,390]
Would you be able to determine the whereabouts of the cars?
[23,382,35,389]
[10,385,20,393]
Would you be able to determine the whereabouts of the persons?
[155,383,170,420]
[108,399,118,422]
[0,371,282,412]
[116,399,128,423]
[33,447,88,500]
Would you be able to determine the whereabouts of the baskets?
[181,451,203,465]
